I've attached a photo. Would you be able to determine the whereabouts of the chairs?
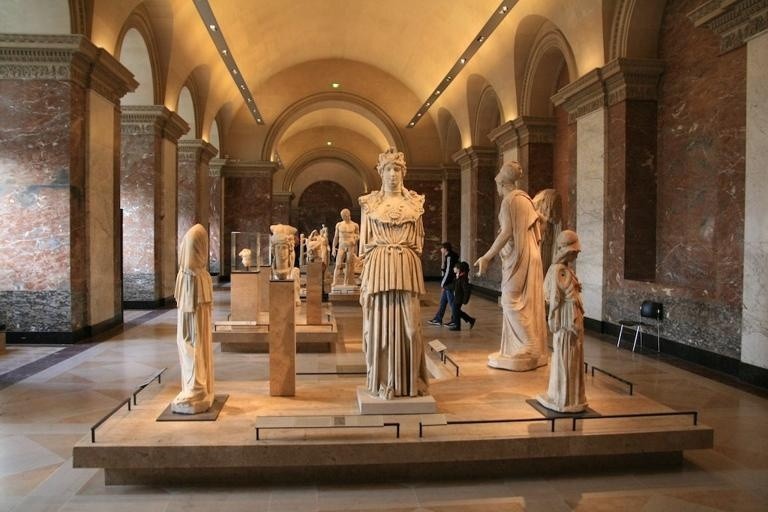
[615,301,662,353]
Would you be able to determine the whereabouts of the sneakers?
[443,322,461,331]
[469,318,475,329]
[427,319,441,326]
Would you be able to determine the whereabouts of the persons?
[269,223,297,280]
[356,146,429,398]
[328,208,360,287]
[472,159,550,365]
[540,229,589,412]
[426,241,460,328]
[173,222,215,405]
[443,261,475,331]
